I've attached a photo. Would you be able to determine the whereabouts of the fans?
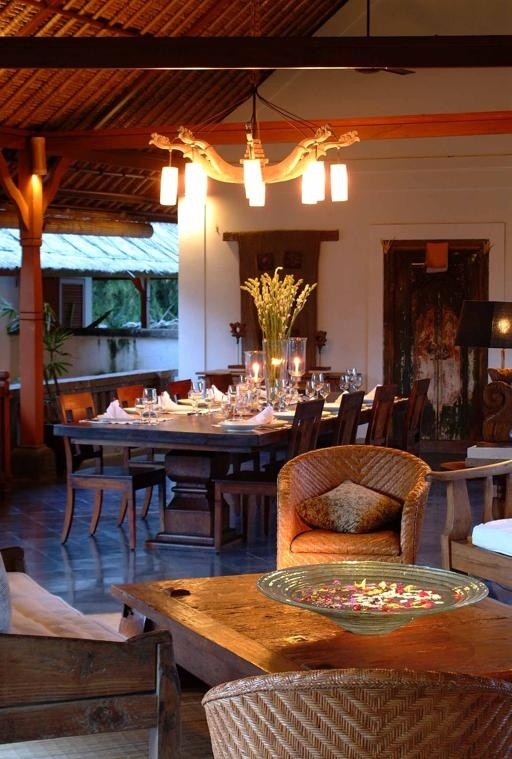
[350,0,415,78]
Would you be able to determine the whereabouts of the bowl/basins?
[256,561,490,636]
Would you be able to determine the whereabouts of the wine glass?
[142,388,157,421]
[187,368,362,421]
[135,397,148,424]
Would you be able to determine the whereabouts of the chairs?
[204,667,511,759]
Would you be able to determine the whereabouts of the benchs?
[0,546,181,759]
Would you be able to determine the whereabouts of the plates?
[219,422,259,432]
[362,399,374,405]
[123,407,138,414]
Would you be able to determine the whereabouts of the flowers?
[238,266,319,382]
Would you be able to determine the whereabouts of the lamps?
[149,67,360,209]
[451,299,512,382]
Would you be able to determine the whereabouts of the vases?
[262,338,294,411]
